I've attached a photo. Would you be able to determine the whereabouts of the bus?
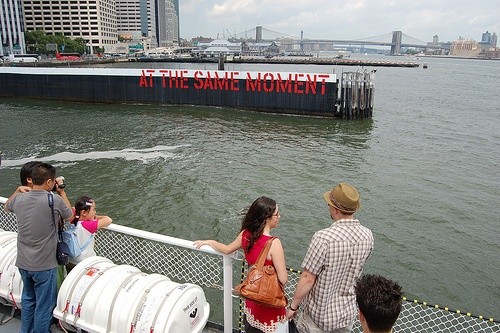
[9,54,39,62]
[56,52,81,61]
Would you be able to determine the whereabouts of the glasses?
[272,211,279,217]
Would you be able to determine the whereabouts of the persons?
[4,161,113,333]
[193,196,288,333]
[286,183,374,333]
[353,273,406,333]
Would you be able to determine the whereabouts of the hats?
[323,183,363,213]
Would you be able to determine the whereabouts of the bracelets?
[290,303,299,311]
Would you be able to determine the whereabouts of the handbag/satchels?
[234,236,289,308]
[56,242,74,265]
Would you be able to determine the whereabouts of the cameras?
[52,176,66,191]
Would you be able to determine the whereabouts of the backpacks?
[63,220,94,257]
